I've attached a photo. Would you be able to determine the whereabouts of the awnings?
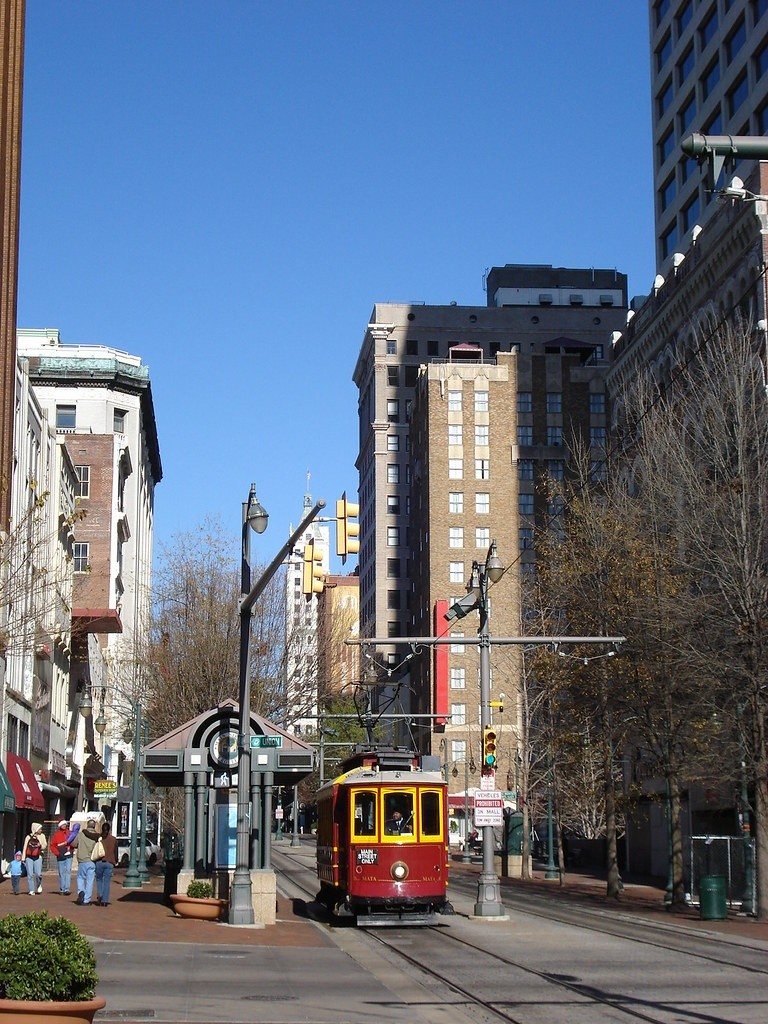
[0,750,46,813]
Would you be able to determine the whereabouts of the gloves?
[3,871,9,876]
[22,861,25,865]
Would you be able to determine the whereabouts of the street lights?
[439,738,448,782]
[363,657,378,748]
[94,704,150,881]
[464,538,505,916]
[78,683,141,888]
[229,483,270,925]
[440,757,476,864]
[709,687,754,911]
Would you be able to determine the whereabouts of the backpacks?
[26,834,41,857]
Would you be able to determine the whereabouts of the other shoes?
[28,890,35,896]
[65,891,71,895]
[97,896,103,902]
[60,892,64,896]
[101,903,108,907]
[77,891,85,905]
[37,884,42,893]
[84,903,89,906]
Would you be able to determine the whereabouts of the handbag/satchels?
[90,834,106,861]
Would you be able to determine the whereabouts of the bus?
[314,752,450,928]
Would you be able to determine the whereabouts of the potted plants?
[170,880,230,919]
[0,908,106,1024]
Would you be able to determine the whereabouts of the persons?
[464,830,478,850]
[388,808,412,833]
[5,819,119,907]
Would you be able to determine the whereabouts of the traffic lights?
[483,729,497,768]
[303,538,324,603]
[278,793,282,804]
[336,490,361,566]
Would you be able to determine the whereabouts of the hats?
[58,815,68,828]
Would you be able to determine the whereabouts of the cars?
[115,837,162,868]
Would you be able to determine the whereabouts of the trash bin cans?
[164,858,182,899]
[700,875,727,920]
